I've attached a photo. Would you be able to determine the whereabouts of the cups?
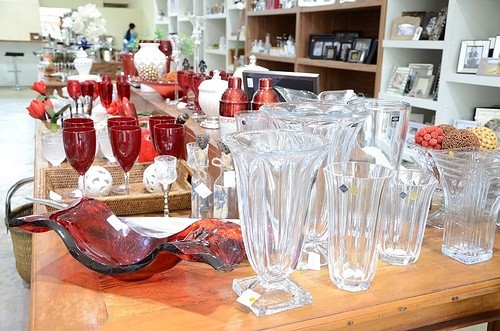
[429,146,500,265]
[222,86,437,317]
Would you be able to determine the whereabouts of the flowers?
[60,4,111,51]
[26,81,73,132]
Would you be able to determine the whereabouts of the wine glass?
[427,148,453,229]
[177,71,229,118]
[42,75,212,218]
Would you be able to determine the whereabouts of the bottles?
[218,77,249,135]
[251,78,279,110]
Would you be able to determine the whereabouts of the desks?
[32,78,500,331]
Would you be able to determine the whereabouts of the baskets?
[40,159,194,218]
[5,177,34,284]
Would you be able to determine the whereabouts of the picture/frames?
[387,63,440,101]
[307,30,379,64]
[391,7,447,40]
[456,36,500,76]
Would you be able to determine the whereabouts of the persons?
[467,47,479,65]
[124,23,137,52]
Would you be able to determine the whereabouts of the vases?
[68,48,102,83]
[134,43,168,81]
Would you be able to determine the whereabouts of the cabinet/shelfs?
[245,0,388,98]
[379,0,500,137]
[154,0,246,72]
